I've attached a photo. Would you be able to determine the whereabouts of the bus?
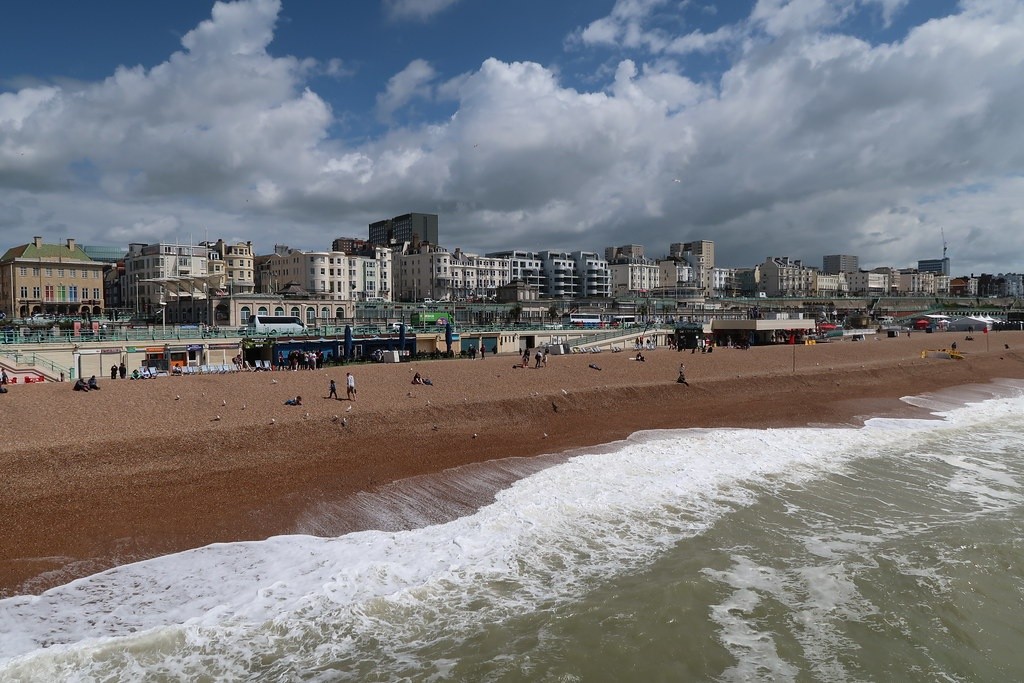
[564,313,602,324]
[248,315,310,335]
[612,315,636,325]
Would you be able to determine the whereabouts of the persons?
[111,364,118,379]
[88,375,100,390]
[346,373,356,401]
[284,396,302,406]
[131,369,142,380]
[74,378,91,392]
[329,379,337,400]
[414,372,433,386]
[0,367,9,385]
[276,344,549,371]
[173,363,183,375]
[141,367,151,379]
[202,324,220,338]
[590,333,751,387]
[119,363,126,379]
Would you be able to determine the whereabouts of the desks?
[29,378,39,383]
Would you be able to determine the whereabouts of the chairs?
[611,346,621,353]
[579,346,600,354]
[633,343,656,351]
[24,375,44,383]
[11,377,17,383]
[140,360,272,380]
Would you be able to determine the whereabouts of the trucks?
[411,311,455,328]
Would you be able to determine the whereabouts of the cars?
[238,325,248,335]
[387,322,414,332]
[424,298,435,303]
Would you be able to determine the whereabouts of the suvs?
[26,313,56,321]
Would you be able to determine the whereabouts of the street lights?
[159,302,167,340]
